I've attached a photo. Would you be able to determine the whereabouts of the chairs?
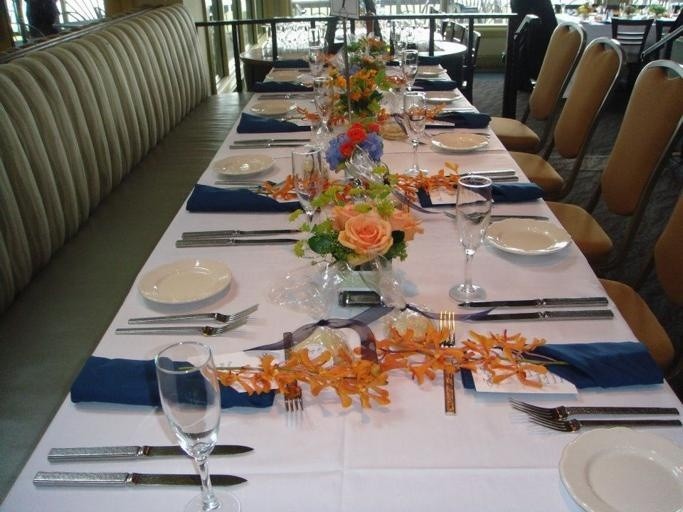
[509,36,621,199]
[442,18,482,105]
[543,59,683,256]
[611,17,653,68]
[656,20,683,57]
[452,23,464,45]
[489,21,586,153]
[598,194,682,372]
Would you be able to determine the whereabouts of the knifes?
[458,296,609,307]
[33,471,246,487]
[229,143,305,148]
[215,178,279,186]
[468,310,614,321]
[46,443,254,462]
[181,229,302,240]
[234,140,309,143]
[450,166,519,183]
[175,237,299,249]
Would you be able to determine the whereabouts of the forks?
[438,310,455,416]
[129,303,256,324]
[528,410,680,433]
[446,208,550,224]
[282,332,303,413]
[116,315,246,336]
[509,399,678,419]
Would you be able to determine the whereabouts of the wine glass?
[154,17,493,91]
[401,91,431,180]
[313,94,332,140]
[449,174,492,301]
[155,341,242,511]
[291,145,327,260]
[560,0,673,22]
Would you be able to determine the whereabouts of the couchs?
[1,5,248,496]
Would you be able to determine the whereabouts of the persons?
[23,0,62,40]
[502,0,559,95]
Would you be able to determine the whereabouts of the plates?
[483,219,572,255]
[214,154,275,175]
[557,426,683,512]
[140,258,231,305]
[249,97,299,115]
[430,131,488,153]
[418,91,460,103]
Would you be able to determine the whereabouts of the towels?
[237,113,298,133]
[187,183,299,214]
[71,354,271,409]
[418,77,454,90]
[441,111,489,129]
[458,340,663,390]
[253,79,314,93]
[422,181,542,205]
[274,58,307,69]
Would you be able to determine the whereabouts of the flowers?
[348,37,390,71]
[302,69,386,126]
[326,119,384,172]
[292,174,423,272]
